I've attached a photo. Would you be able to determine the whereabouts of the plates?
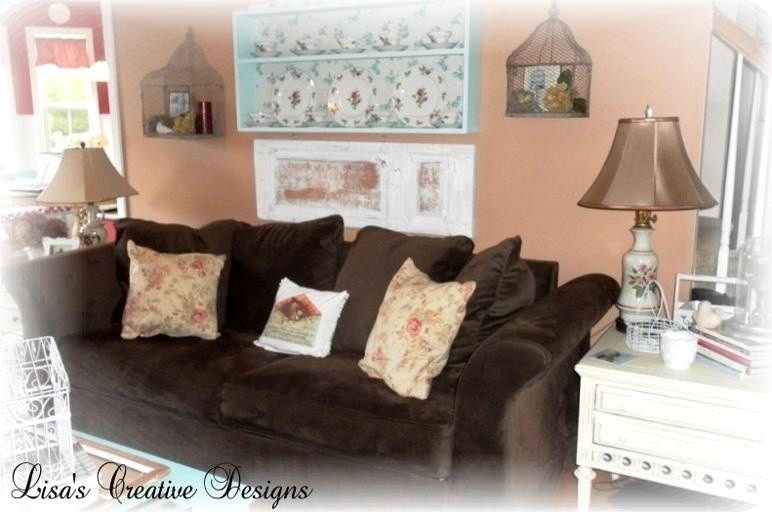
[391,64,441,125]
[364,121,396,128]
[371,44,408,50]
[270,69,316,126]
[433,122,462,129]
[331,48,365,53]
[421,42,457,49]
[249,51,281,57]
[245,122,272,128]
[302,121,331,127]
[292,48,324,56]
[328,67,376,127]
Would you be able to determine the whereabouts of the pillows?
[113,215,536,401]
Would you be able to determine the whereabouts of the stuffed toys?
[10,211,69,254]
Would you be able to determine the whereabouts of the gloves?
[625,280,682,355]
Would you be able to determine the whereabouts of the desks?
[575,319,768,510]
[70,429,258,511]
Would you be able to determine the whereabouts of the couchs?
[3,239,621,511]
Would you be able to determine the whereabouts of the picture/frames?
[72,435,170,510]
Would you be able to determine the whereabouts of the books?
[686,322,765,375]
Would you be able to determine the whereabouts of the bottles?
[197,101,213,134]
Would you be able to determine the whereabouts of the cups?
[305,111,327,121]
[380,35,400,45]
[440,112,462,124]
[337,38,359,48]
[370,111,392,121]
[249,112,269,121]
[256,40,277,51]
[659,332,697,368]
[427,32,451,42]
[296,39,320,49]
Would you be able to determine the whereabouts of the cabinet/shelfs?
[230,4,482,135]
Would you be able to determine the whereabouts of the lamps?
[577,104,719,333]
[36,142,139,250]
